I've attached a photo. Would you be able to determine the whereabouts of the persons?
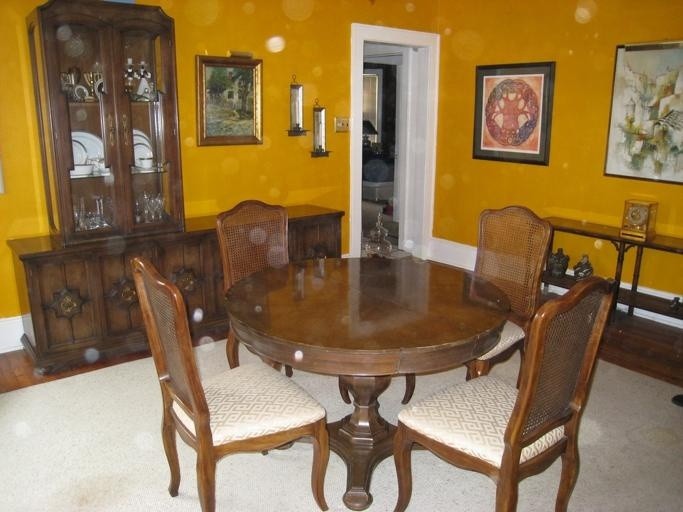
[573,255,592,281]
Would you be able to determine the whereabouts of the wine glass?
[134,190,165,225]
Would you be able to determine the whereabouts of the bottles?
[365,211,393,257]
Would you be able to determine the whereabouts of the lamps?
[363,119,379,152]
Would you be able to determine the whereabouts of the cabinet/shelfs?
[535,216,683,389]
[6,203,346,375]
[24,1,186,248]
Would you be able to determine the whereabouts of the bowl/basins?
[70,164,94,179]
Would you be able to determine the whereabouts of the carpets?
[1,336,682,512]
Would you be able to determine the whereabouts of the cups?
[71,194,114,232]
[89,159,111,176]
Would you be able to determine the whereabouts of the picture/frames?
[193,55,265,147]
[602,39,683,185]
[470,61,555,165]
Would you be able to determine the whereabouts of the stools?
[362,180,393,204]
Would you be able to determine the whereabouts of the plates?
[70,131,105,165]
[133,129,155,170]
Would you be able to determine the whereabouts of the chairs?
[400,202,555,406]
[124,255,337,512]
[391,275,621,512]
[215,198,295,382]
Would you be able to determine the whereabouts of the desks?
[222,249,512,512]
